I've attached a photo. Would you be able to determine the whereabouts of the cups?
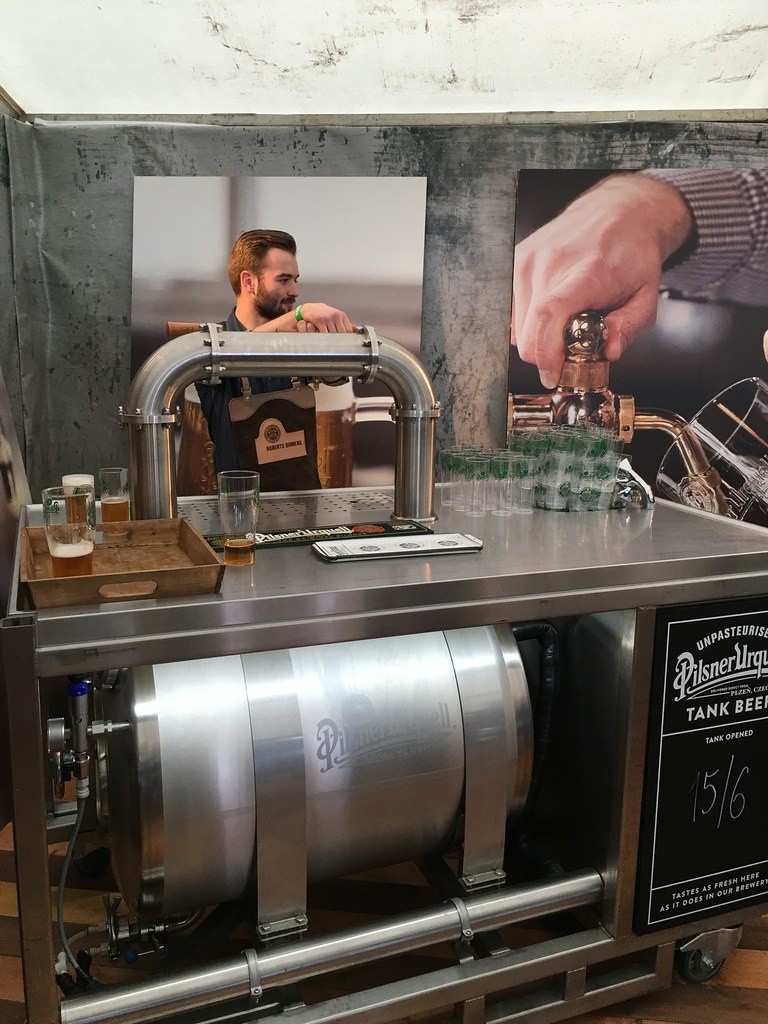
[42,486,95,577]
[218,470,260,567]
[440,422,625,517]
[61,474,95,485]
[653,377,768,529]
[99,468,130,522]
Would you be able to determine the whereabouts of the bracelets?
[295,305,303,320]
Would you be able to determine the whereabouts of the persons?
[194,230,357,492]
[511,166,768,388]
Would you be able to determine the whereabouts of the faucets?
[507,310,731,519]
